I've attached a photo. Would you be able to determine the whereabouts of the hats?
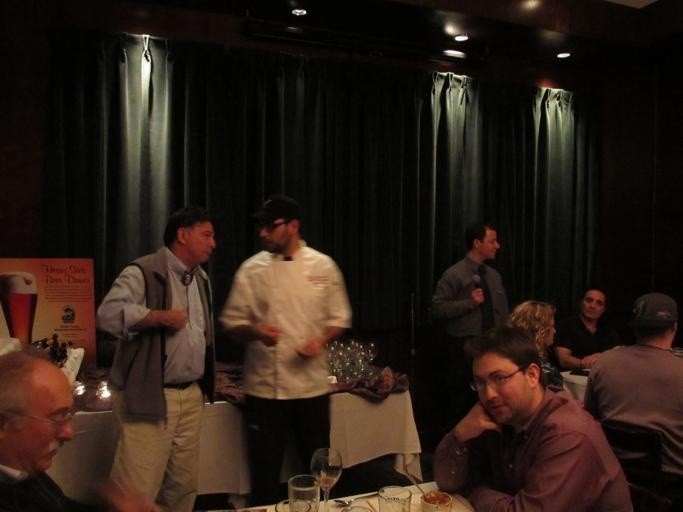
[247,194,301,225]
[626,292,679,323]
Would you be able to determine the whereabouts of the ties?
[477,265,498,351]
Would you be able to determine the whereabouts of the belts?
[163,380,195,391]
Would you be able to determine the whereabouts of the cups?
[419,490,453,512]
[275,447,413,512]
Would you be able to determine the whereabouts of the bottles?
[36,331,74,369]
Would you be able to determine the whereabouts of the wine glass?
[325,339,380,387]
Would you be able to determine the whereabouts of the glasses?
[7,409,78,428]
[469,367,527,392]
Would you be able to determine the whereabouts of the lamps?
[93,376,112,400]
[73,380,87,396]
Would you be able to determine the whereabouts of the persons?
[95,206,218,511]
[434,323,636,512]
[218,194,353,505]
[0,344,163,511]
[507,300,567,395]
[552,288,621,370]
[583,293,683,506]
[431,221,511,354]
